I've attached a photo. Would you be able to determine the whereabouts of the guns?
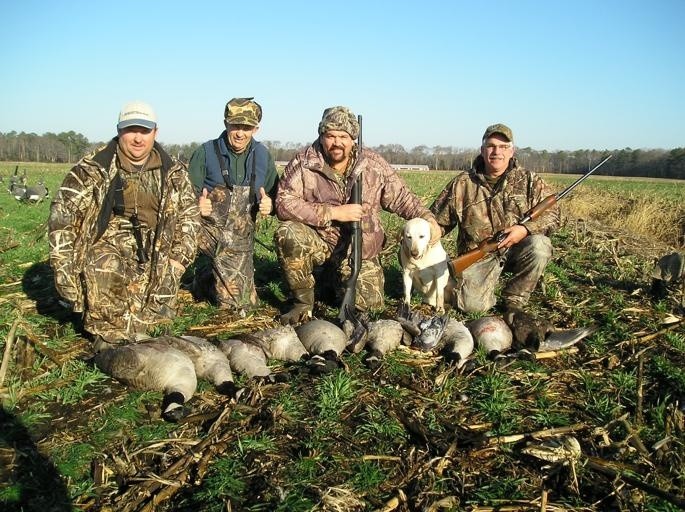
[337,115,364,323]
[446,154,614,280]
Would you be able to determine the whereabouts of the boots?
[277,289,315,325]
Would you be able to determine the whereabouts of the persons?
[186,98,279,312]
[48,101,200,354]
[273,108,441,326]
[396,124,561,308]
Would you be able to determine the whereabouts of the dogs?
[399,216,450,316]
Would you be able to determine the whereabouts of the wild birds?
[7,164,50,208]
[351,299,603,369]
[81,318,355,423]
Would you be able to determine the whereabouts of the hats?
[482,124,514,141]
[319,107,359,139]
[117,104,157,130]
[224,97,262,127]
